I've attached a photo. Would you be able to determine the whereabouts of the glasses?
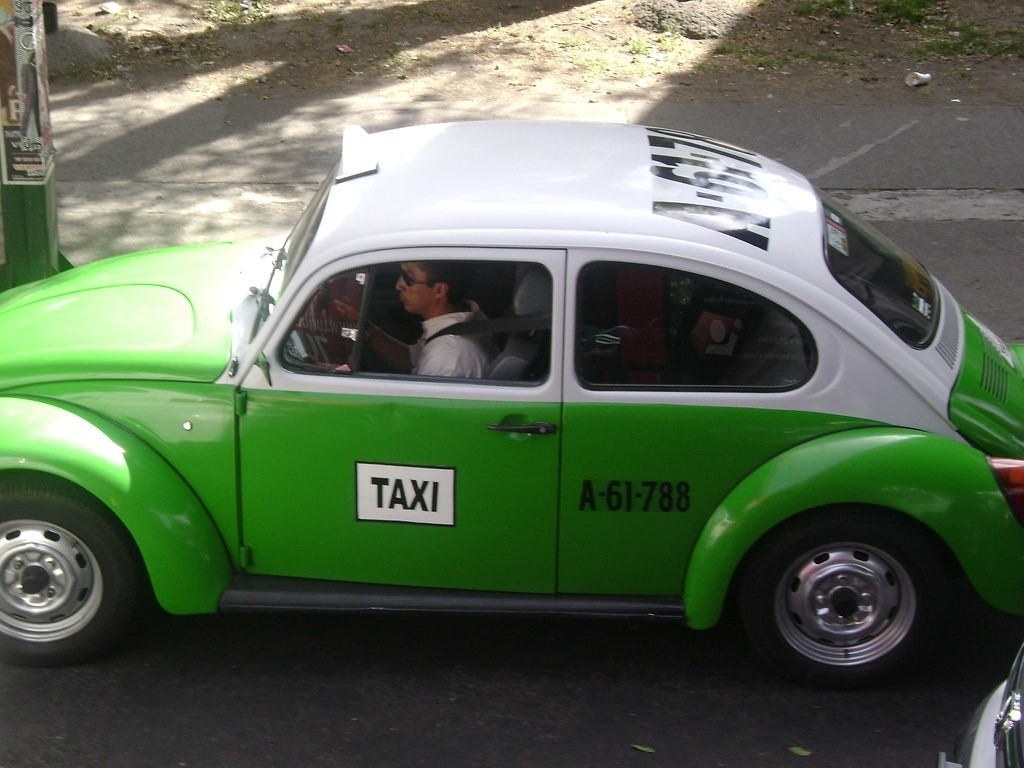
[400,269,443,287]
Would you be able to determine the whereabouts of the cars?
[0,121,1024,695]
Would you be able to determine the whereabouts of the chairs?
[488,261,552,381]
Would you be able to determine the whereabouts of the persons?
[581,278,753,385]
[315,260,493,378]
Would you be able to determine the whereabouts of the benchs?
[723,293,807,388]
